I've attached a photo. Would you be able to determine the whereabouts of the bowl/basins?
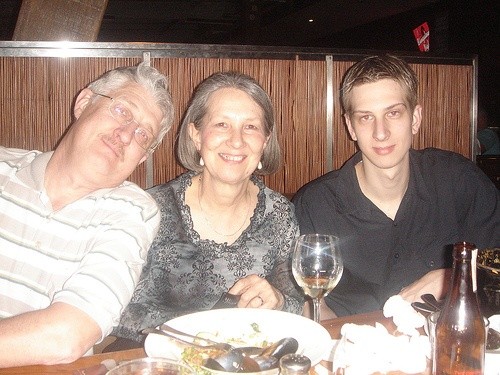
[476,247,500,280]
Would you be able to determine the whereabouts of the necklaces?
[198,179,252,237]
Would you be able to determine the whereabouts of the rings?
[256,295,264,306]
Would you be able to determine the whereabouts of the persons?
[0,59,175,369]
[477,106,500,155]
[103,71,302,354]
[289,52,500,322]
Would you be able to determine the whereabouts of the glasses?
[94,94,155,151]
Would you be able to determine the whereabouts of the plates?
[144,308,331,375]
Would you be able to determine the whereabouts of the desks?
[0,311,432,375]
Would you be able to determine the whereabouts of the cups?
[428,312,490,372]
[105,356,195,375]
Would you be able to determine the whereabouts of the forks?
[142,324,236,352]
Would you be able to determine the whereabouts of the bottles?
[279,353,311,375]
[432,241,486,375]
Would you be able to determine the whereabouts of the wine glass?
[292,234,344,323]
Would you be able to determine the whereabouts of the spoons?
[206,337,299,373]
